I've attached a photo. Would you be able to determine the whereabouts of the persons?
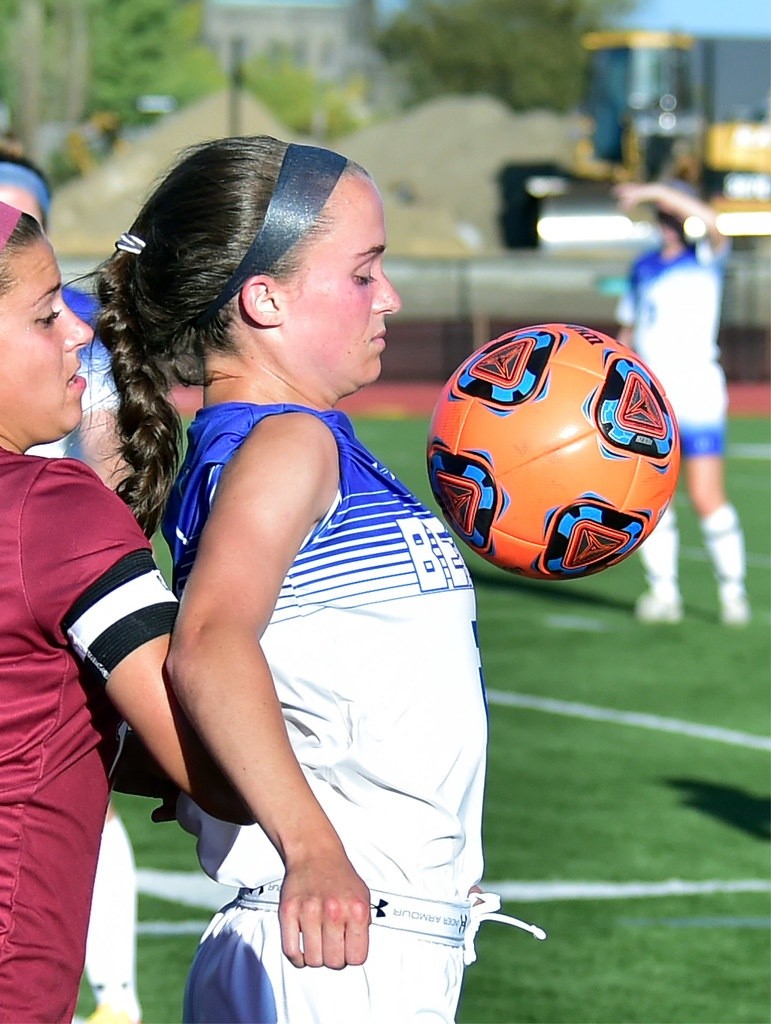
[611,149,753,626]
[85,135,499,1024]
[1,145,255,1024]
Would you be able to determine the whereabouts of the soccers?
[426,320,682,582]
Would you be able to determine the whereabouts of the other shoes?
[84,1004,141,1024]
[718,583,749,624]
[633,593,684,623]
